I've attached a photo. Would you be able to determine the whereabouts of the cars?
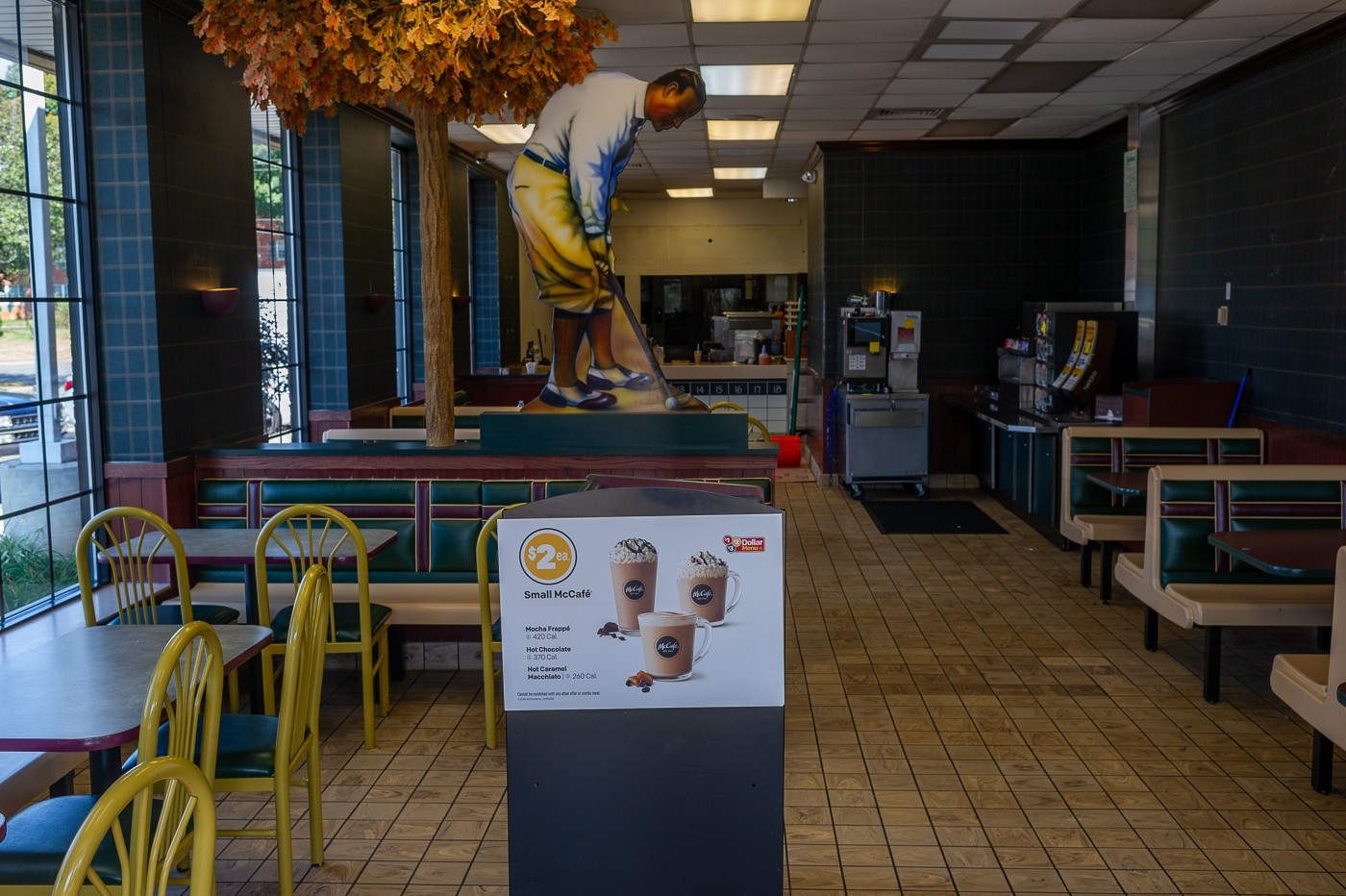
[57,376,76,434]
[261,363,292,435]
[0,391,39,444]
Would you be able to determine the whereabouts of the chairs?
[0,504,392,895]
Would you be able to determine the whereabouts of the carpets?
[860,499,1011,535]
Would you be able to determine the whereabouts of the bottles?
[758,344,770,365]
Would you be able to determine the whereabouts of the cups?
[694,351,702,364]
[846,295,870,306]
[609,552,658,636]
[675,563,743,628]
[636,612,713,682]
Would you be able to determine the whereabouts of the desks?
[95,528,399,712]
[1,624,277,791]
[1084,469,1150,497]
[1205,525,1345,577]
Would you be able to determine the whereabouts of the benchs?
[166,405,1346,796]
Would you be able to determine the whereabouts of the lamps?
[802,171,816,182]
[452,296,472,310]
[203,288,238,316]
[365,295,390,313]
